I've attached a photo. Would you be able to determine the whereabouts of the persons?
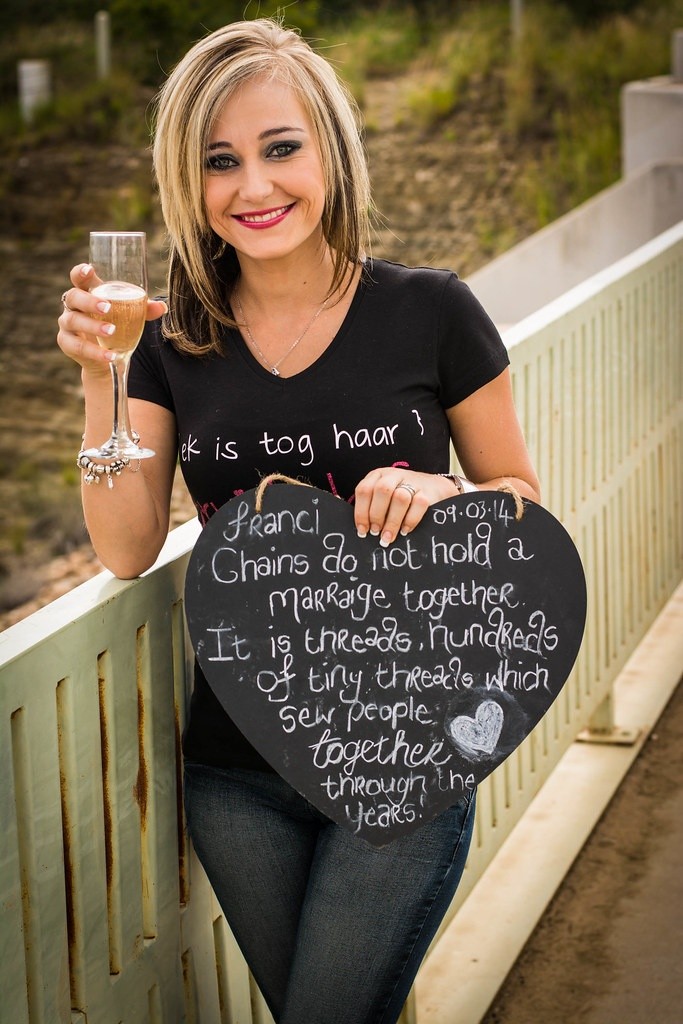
[56,18,543,1024]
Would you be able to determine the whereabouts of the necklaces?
[234,282,337,376]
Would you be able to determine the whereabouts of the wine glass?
[80,231,156,460]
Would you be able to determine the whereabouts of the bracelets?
[73,429,138,490]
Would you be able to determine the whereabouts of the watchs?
[435,473,479,497]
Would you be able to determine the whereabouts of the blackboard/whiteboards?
[182,483,589,851]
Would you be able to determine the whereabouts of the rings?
[397,481,416,498]
[61,289,77,312]
